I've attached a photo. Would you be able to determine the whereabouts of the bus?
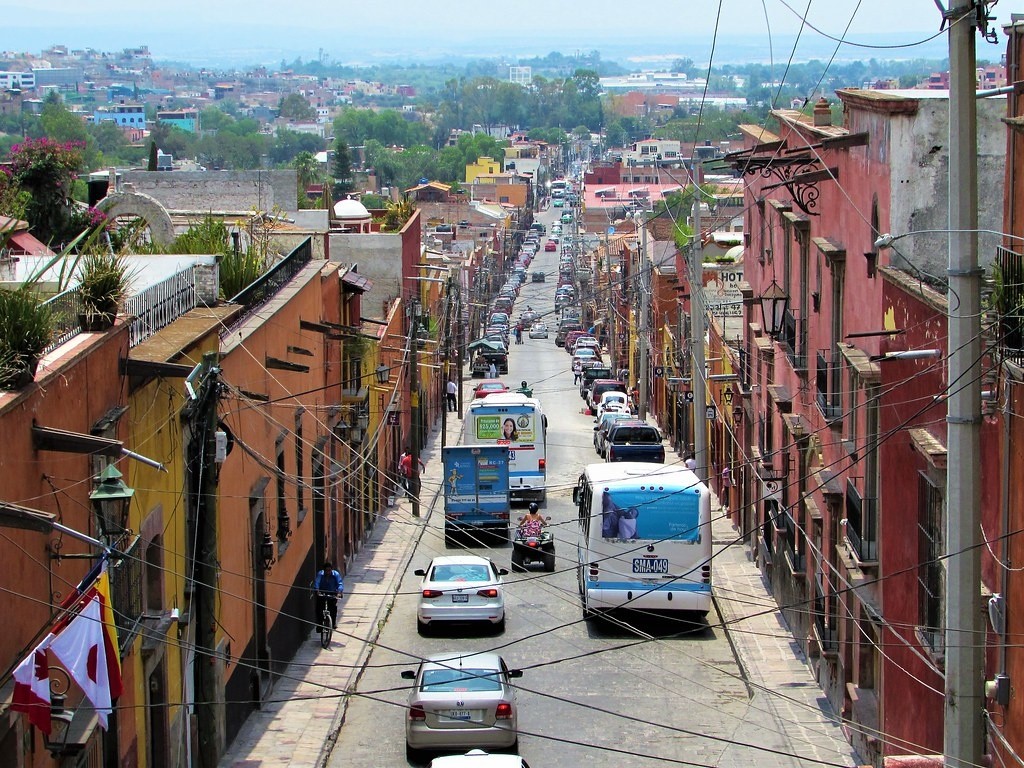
[571,462,712,620]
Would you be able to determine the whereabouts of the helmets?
[529,503,538,512]
[522,381,527,388]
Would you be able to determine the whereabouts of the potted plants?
[0,232,148,398]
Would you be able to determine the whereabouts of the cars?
[414,556,509,631]
[427,748,532,768]
[469,165,669,467]
[400,652,523,760]
[473,381,510,401]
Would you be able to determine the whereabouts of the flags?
[10,544,125,735]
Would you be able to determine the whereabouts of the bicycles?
[316,592,342,649]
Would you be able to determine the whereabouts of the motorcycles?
[510,517,556,570]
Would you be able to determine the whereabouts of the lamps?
[376,363,391,384]
[757,278,792,343]
[383,401,402,425]
[416,323,429,350]
[253,529,276,571]
[723,388,734,405]
[732,406,744,427]
[276,506,293,544]
[41,665,76,761]
[327,409,369,446]
[47,464,135,560]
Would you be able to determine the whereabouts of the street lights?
[666,374,710,488]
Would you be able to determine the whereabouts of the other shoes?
[332,625,337,629]
[317,627,323,632]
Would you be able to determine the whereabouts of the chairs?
[436,571,453,581]
[521,520,541,536]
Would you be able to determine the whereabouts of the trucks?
[464,392,548,502]
[442,444,515,544]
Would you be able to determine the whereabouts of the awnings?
[340,271,375,295]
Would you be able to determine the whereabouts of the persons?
[628,387,639,411]
[470,566,484,574]
[447,379,458,412]
[625,439,632,445]
[513,322,522,342]
[398,447,425,502]
[618,364,630,385]
[527,305,532,311]
[573,361,582,385]
[501,418,518,441]
[518,503,549,533]
[517,381,533,392]
[721,464,732,509]
[685,451,696,472]
[488,358,500,377]
[438,566,452,574]
[315,562,343,632]
[604,493,640,540]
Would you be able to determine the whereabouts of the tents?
[404,182,451,195]
[467,339,498,370]
